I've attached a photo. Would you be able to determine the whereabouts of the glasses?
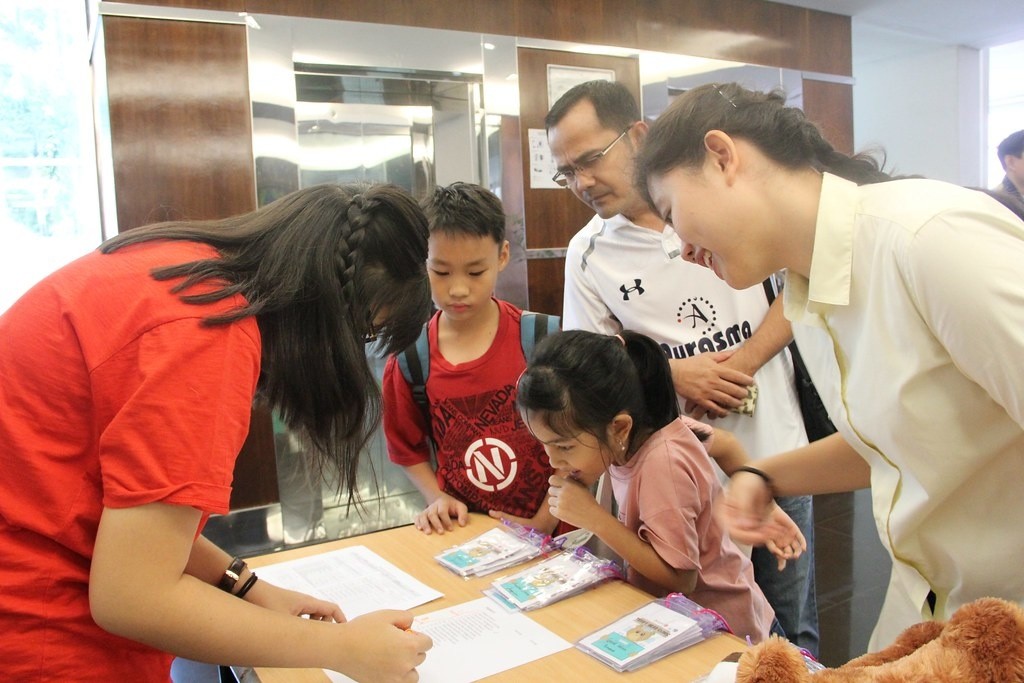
[551,126,631,188]
[361,308,377,343]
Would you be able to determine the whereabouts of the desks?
[212,508,759,683]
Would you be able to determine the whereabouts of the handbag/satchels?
[788,342,838,443]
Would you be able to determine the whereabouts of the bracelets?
[237,572,258,598]
[730,466,769,481]
[218,557,247,592]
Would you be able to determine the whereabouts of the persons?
[543,79,820,667]
[632,82,1024,653]
[381,180,578,539]
[516,329,787,642]
[994,129,1024,221]
[0,182,432,683]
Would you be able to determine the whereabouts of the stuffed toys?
[734,595,1024,683]
